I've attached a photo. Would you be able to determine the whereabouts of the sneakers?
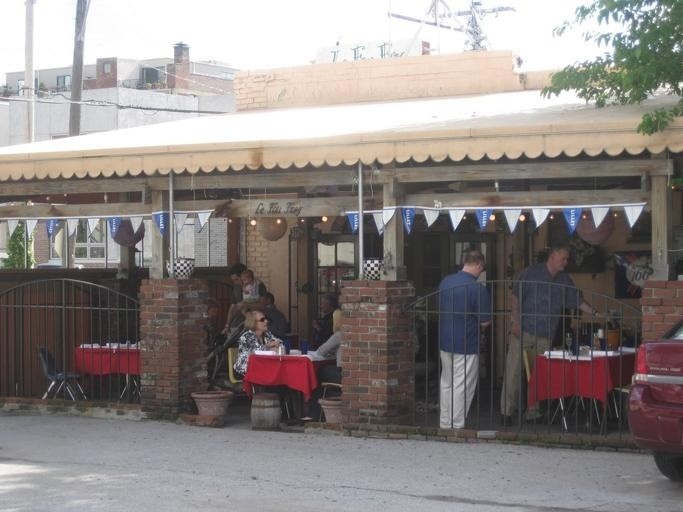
[526,409,545,421]
[502,414,512,425]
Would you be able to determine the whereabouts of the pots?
[593,330,620,350]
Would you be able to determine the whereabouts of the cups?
[283,340,290,354]
[301,340,308,355]
[598,328,606,350]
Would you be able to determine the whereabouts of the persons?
[207,263,343,421]
[436,250,492,429]
[500,240,617,426]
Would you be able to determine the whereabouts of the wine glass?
[565,333,573,356]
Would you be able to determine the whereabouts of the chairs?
[227,347,290,420]
[319,382,343,422]
[600,384,631,437]
[521,349,601,433]
[37,347,93,402]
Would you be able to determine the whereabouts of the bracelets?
[591,308,600,319]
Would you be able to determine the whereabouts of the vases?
[362,256,383,280]
[317,396,342,423]
[164,257,195,281]
[190,390,233,427]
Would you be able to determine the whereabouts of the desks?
[69,345,141,401]
[249,350,338,427]
[535,348,638,432]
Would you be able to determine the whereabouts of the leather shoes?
[295,417,313,422]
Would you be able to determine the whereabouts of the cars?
[627,320,681,483]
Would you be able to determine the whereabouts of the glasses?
[257,317,266,322]
